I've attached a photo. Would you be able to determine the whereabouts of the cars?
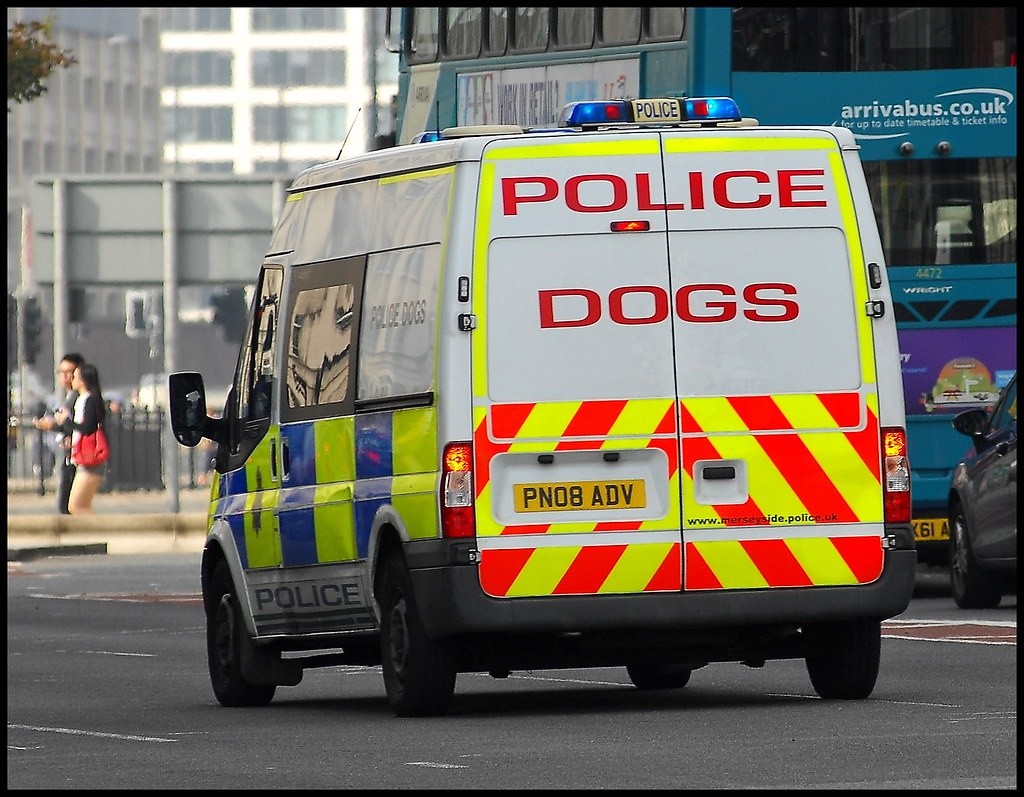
[944,369,1018,619]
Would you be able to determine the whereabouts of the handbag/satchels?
[75,394,108,466]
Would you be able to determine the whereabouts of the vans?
[167,95,918,720]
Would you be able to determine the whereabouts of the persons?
[36,353,109,515]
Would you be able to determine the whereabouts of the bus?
[364,0,1023,570]
